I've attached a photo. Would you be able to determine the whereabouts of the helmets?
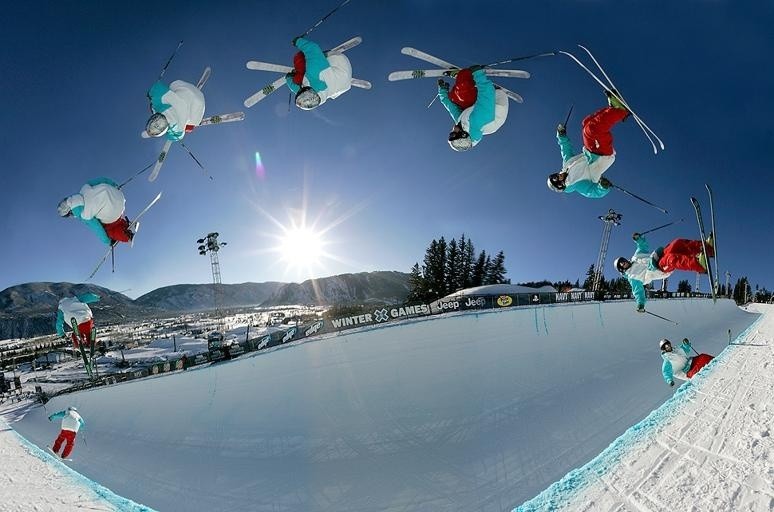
[146,113,169,138]
[547,174,566,192]
[65,288,76,297]
[295,86,321,111]
[614,257,631,274]
[448,132,472,152]
[69,407,77,411]
[57,198,71,217]
[659,339,669,349]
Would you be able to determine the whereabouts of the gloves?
[292,37,299,46]
[286,72,295,77]
[437,80,449,89]
[636,304,645,313]
[470,65,481,72]
[558,124,566,135]
[683,338,689,344]
[632,233,641,240]
[600,177,612,189]
[668,381,674,386]
[110,240,116,245]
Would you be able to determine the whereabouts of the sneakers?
[443,71,459,79]
[75,348,90,357]
[126,222,139,247]
[706,232,713,248]
[695,246,707,271]
[604,88,631,116]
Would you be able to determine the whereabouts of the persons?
[545,89,631,200]
[52,287,103,357]
[47,405,85,461]
[433,63,510,155]
[612,228,715,313]
[139,80,206,142]
[284,35,353,113]
[55,179,139,249]
[658,336,714,386]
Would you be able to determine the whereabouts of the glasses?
[664,342,670,348]
[619,259,628,266]
[551,173,559,184]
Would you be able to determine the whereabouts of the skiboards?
[90,192,162,280]
[244,35,372,108]
[689,183,719,304]
[559,45,665,155]
[726,329,732,345]
[71,317,96,380]
[140,67,246,183]
[46,445,73,463]
[387,47,530,104]
